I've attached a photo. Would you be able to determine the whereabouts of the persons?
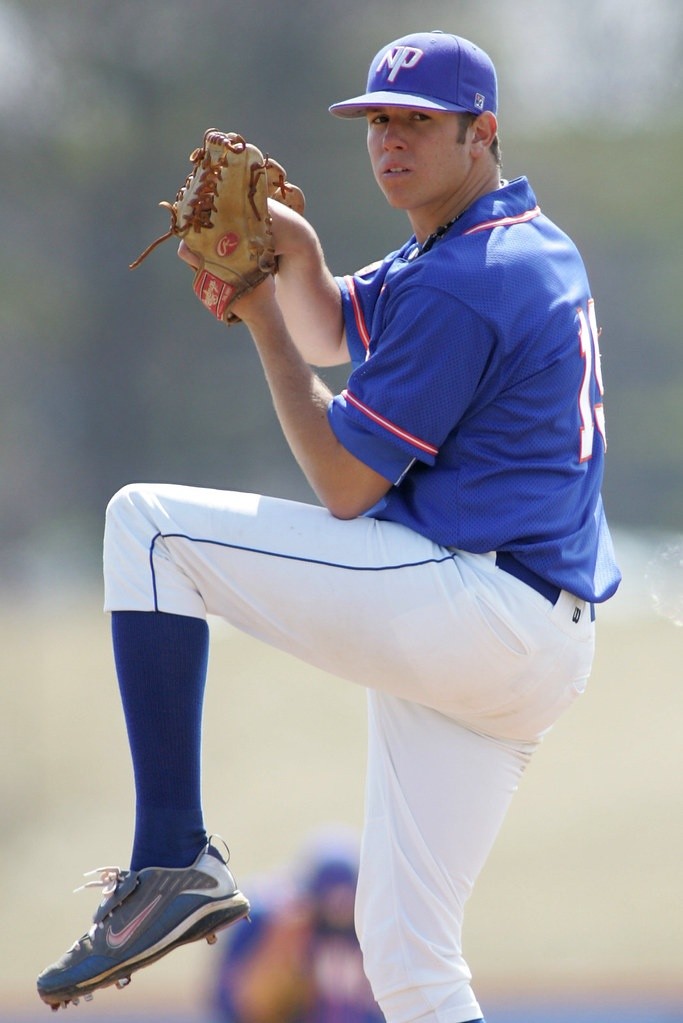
[214,857,388,1023]
[35,29,625,1023]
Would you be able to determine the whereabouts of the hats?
[328,30,497,118]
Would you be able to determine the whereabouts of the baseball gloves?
[158,125,307,328]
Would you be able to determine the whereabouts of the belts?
[496,553,583,624]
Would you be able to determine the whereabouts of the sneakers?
[38,840,251,1013]
